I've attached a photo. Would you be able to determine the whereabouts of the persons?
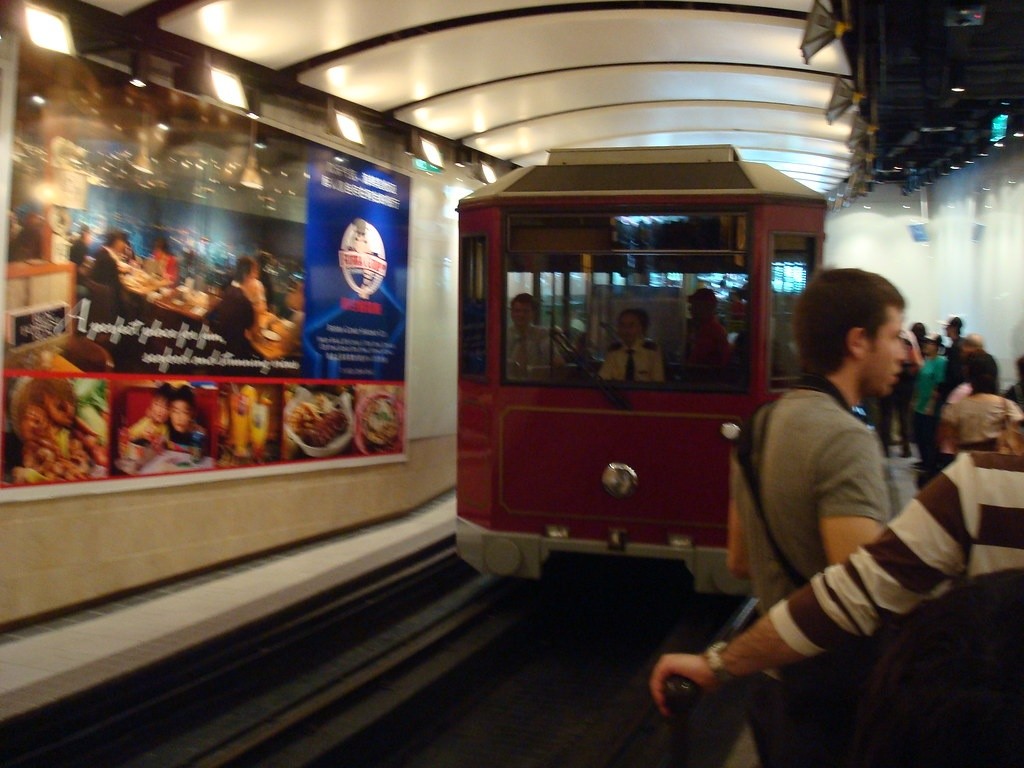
[7,206,307,374]
[596,312,665,383]
[650,450,1024,719]
[122,380,205,455]
[13,375,110,466]
[684,286,729,367]
[506,293,564,379]
[850,570,1024,768]
[724,267,909,768]
[874,316,1024,488]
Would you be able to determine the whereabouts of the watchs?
[703,641,734,686]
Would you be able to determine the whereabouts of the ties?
[624,349,635,381]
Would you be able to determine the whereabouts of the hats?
[924,334,942,345]
[687,288,716,306]
[939,315,962,329]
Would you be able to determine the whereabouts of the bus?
[452,144,830,601]
